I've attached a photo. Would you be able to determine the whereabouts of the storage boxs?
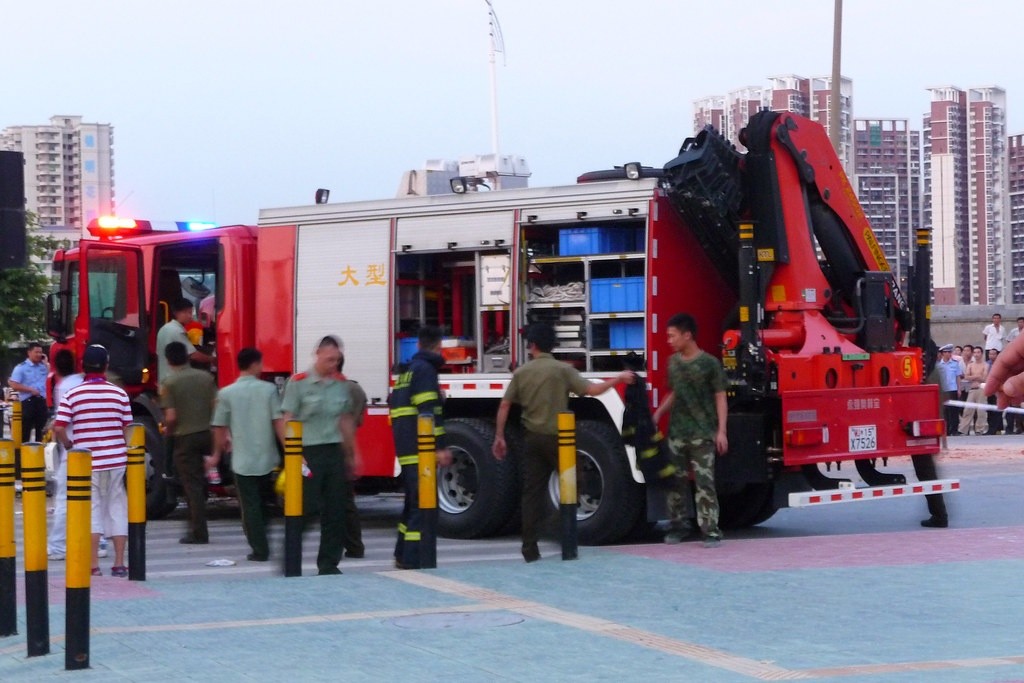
[591,277,647,312]
[397,338,420,364]
[559,226,635,256]
[608,320,644,351]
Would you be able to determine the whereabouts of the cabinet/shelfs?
[517,212,648,379]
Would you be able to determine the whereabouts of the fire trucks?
[41,107,949,546]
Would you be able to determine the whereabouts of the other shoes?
[318,567,342,574]
[47,547,64,560]
[179,534,208,545]
[664,527,690,542]
[247,552,267,560]
[95,540,108,557]
[704,534,719,547]
[345,550,364,557]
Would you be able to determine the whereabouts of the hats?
[939,344,953,351]
[83,343,108,367]
[524,321,556,348]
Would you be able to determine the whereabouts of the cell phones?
[41,355,44,359]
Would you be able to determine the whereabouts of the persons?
[984,333,1024,410]
[156,298,216,509]
[1007,317,1024,343]
[653,312,729,549]
[386,323,451,569]
[8,342,48,492]
[55,344,128,577]
[158,342,217,544]
[982,313,1005,361]
[205,347,284,561]
[278,335,368,577]
[47,349,108,560]
[492,321,634,561]
[935,344,1024,436]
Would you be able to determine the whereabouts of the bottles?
[302,464,313,479]
[203,456,221,484]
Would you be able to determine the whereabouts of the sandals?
[111,565,127,576]
[91,566,102,576]
